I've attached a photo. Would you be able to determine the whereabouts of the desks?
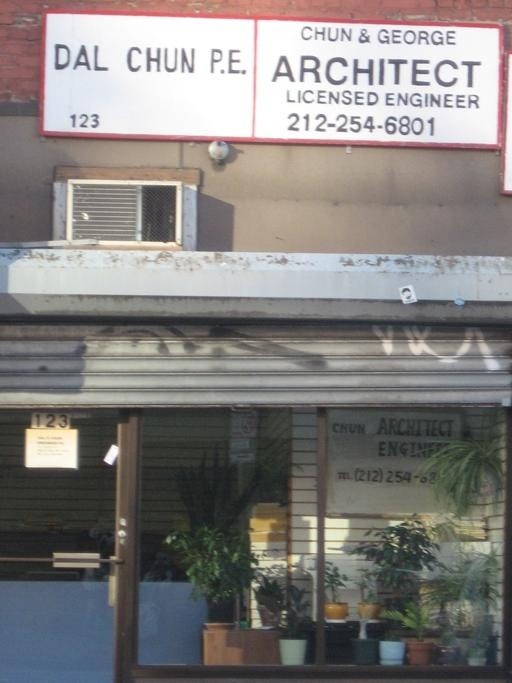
[0,579,208,681]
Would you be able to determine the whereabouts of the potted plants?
[252,575,309,665]
[255,573,282,625]
[161,525,281,666]
[321,515,494,670]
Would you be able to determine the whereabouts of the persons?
[402,286,413,302]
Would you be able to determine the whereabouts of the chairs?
[25,568,81,581]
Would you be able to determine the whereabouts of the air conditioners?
[67,177,185,251]
